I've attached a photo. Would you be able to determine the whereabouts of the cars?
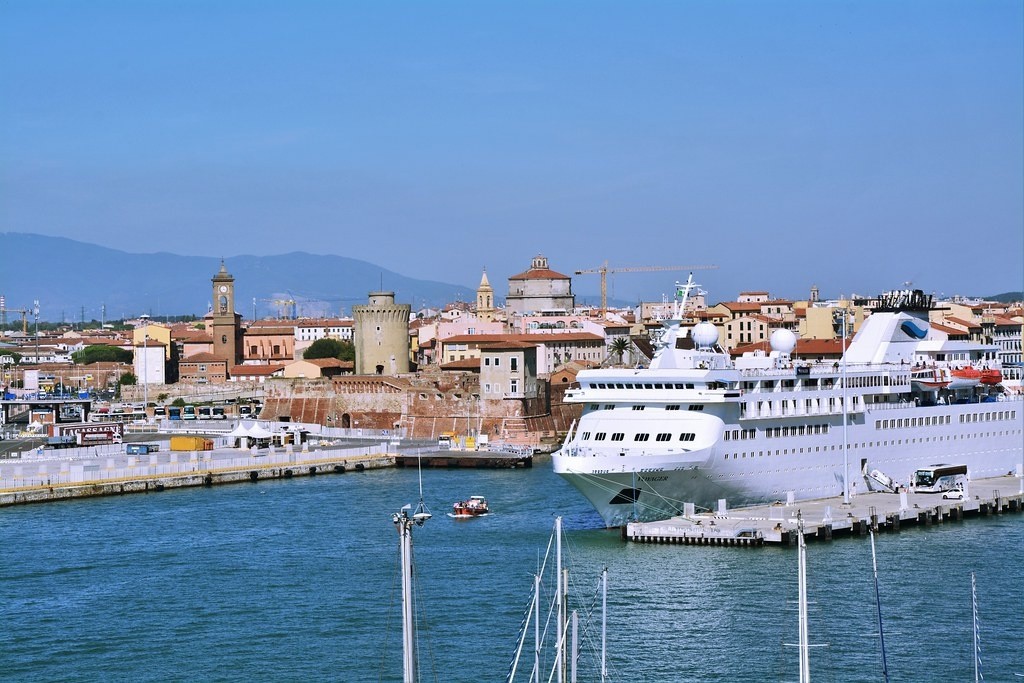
[67,411,80,417]
[96,399,132,413]
[102,388,115,393]
[321,442,330,447]
[941,488,970,500]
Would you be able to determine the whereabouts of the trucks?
[437,435,451,449]
[77,430,123,445]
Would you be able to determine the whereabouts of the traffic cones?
[136,453,141,462]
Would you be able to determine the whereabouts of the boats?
[453,496,489,515]
[550,271,1024,529]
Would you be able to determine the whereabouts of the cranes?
[574,260,720,319]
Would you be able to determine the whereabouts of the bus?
[913,463,970,493]
[154,407,166,419]
[168,407,180,420]
[199,406,211,420]
[240,406,251,418]
[0,430,21,439]
[183,406,196,420]
[212,407,224,419]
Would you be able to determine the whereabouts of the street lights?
[17,379,21,400]
[472,393,480,448]
[458,407,469,437]
[141,314,150,408]
[96,362,99,389]
